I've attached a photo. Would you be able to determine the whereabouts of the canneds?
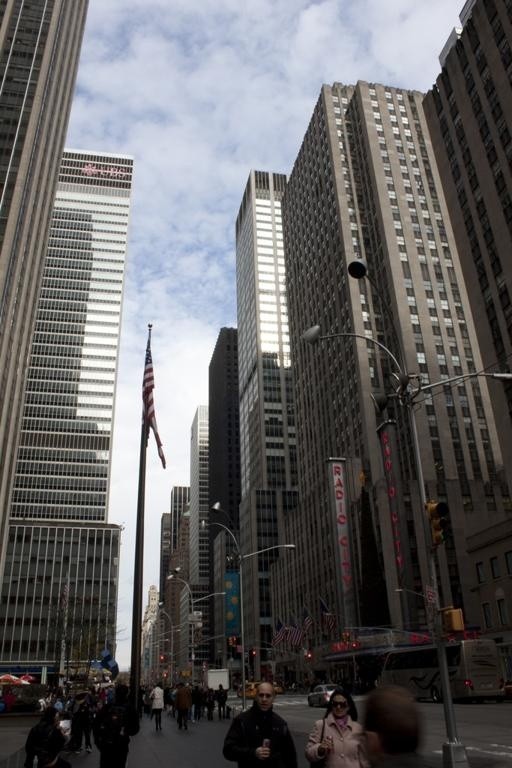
[326,736,334,755]
[262,739,270,748]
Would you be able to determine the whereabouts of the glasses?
[331,701,347,708]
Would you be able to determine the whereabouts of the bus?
[377,639,506,703]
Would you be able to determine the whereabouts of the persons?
[304,689,371,768]
[362,685,442,768]
[223,682,298,768]
[24,682,227,768]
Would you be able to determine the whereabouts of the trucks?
[203,669,230,692]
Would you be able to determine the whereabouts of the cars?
[237,681,260,700]
[273,681,285,695]
[308,684,343,707]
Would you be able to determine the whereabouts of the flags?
[299,606,314,639]
[271,618,287,648]
[286,614,302,646]
[142,339,166,469]
[320,599,337,635]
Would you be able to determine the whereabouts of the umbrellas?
[0,674,35,685]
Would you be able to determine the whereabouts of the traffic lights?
[428,499,454,546]
[251,647,256,656]
[303,648,312,660]
[341,631,360,653]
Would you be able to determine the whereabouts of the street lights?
[200,501,296,713]
[301,259,512,768]
[394,589,432,633]
[155,602,187,687]
[166,566,227,718]
